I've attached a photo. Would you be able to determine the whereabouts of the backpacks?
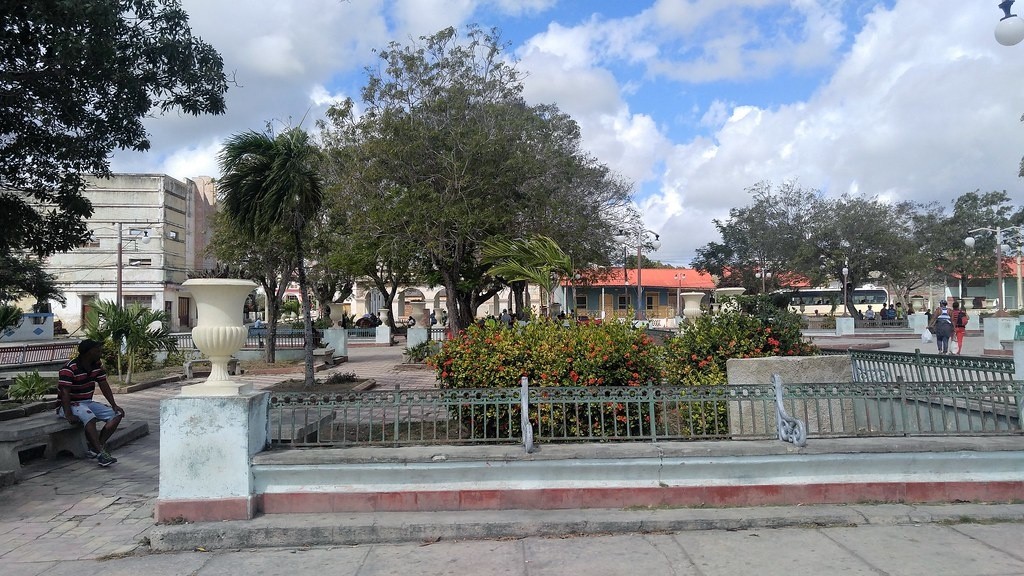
[956,311,968,328]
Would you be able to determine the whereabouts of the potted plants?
[911,295,924,313]
[181,262,258,382]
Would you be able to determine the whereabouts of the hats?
[78,339,105,355]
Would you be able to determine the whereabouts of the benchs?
[270,410,337,443]
[183,358,241,379]
[313,348,335,364]
[0,411,89,482]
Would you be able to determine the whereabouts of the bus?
[786,283,890,315]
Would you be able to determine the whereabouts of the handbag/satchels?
[921,329,933,343]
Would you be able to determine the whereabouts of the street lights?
[86,222,150,309]
[614,227,661,319]
[965,226,1024,318]
[840,266,850,318]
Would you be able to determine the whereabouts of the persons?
[815,310,828,318]
[370,312,381,327]
[803,297,873,305]
[857,302,915,326]
[479,308,517,332]
[538,309,575,330]
[925,298,969,356]
[342,310,349,328]
[406,316,415,329]
[710,294,715,303]
[429,310,447,326]
[57,339,125,467]
[301,305,332,349]
[255,318,261,328]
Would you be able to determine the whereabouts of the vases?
[962,298,975,309]
[680,292,706,326]
[327,303,345,329]
[378,308,390,327]
[434,308,444,327]
[410,302,426,329]
[715,287,746,314]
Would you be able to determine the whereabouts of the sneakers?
[86,449,111,459]
[97,450,117,467]
